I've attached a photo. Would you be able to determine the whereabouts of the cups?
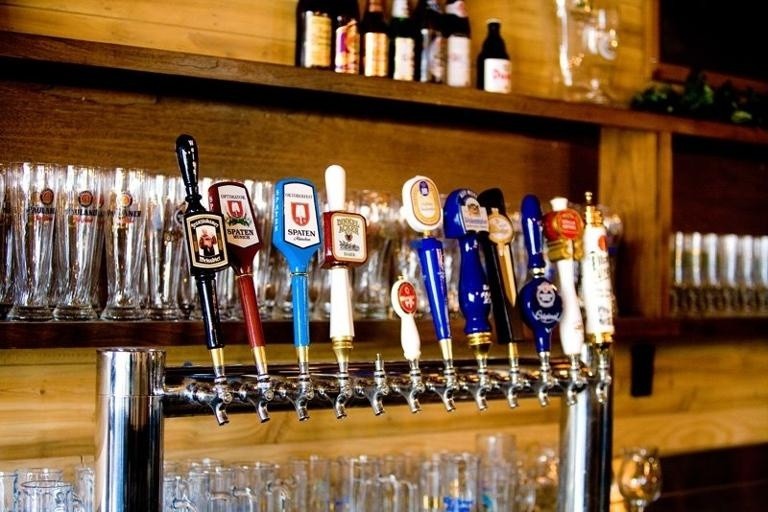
[3,431,559,510]
[2,161,466,321]
[617,446,662,511]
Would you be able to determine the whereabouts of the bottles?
[476,19,514,94]
[331,0,362,75]
[412,0,444,84]
[444,0,472,87]
[389,0,418,82]
[361,0,390,77]
[295,0,333,70]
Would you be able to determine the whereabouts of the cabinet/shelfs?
[0,0,768,512]
